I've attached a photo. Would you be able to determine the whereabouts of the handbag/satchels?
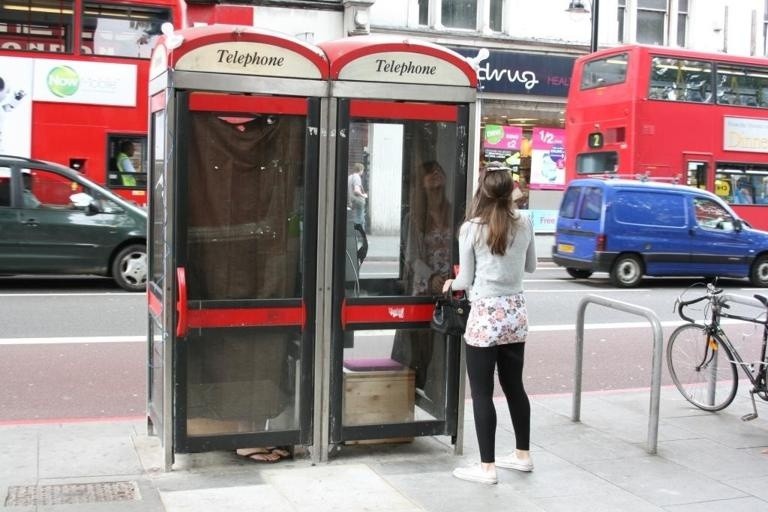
[430,282,472,335]
[351,190,366,208]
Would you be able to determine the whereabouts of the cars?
[0,153,148,294]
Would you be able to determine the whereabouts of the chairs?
[648,85,766,108]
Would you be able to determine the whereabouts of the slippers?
[235,446,292,462]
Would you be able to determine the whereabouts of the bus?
[564,46,768,236]
[0,0,190,213]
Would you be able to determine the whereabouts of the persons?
[112,141,138,187]
[735,178,754,204]
[401,160,455,407]
[234,419,290,462]
[347,163,369,231]
[21,173,75,210]
[441,162,537,484]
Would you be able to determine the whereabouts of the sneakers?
[452,464,498,485]
[499,456,536,472]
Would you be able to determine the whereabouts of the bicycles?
[662,270,767,425]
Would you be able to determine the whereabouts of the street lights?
[566,0,600,53]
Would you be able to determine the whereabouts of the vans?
[549,170,767,286]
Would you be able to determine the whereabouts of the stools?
[341,356,417,446]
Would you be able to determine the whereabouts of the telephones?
[345,220,368,282]
